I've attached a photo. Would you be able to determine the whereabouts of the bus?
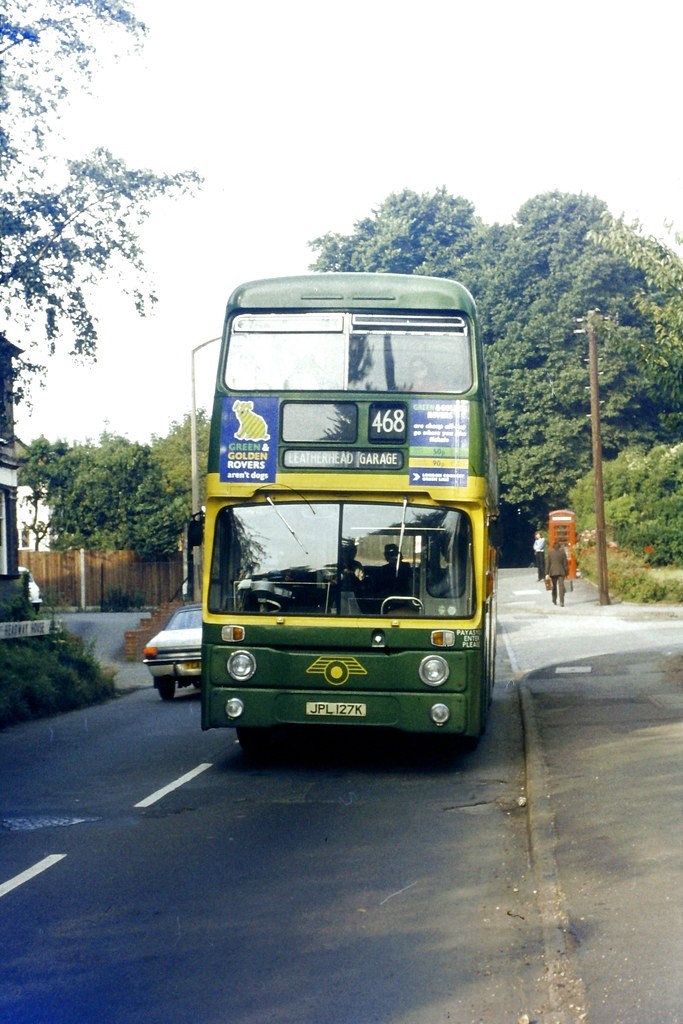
[183,271,502,757]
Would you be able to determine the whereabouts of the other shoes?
[560,602,564,607]
[553,600,557,605]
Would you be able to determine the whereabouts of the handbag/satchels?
[544,575,554,591]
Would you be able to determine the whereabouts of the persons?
[402,355,446,391]
[427,542,466,597]
[532,530,546,582]
[375,544,412,581]
[340,535,365,581]
[545,542,570,607]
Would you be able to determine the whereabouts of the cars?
[142,600,203,701]
[17,566,43,614]
